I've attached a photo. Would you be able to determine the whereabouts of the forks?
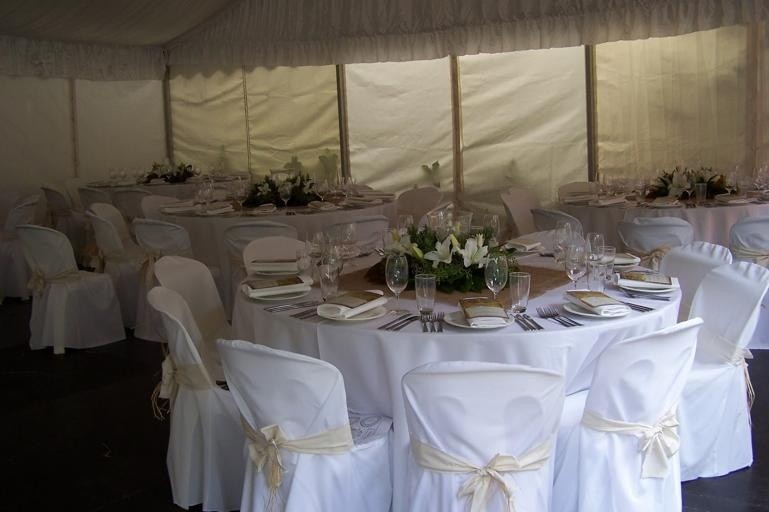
[536,305,584,327]
[419,310,445,333]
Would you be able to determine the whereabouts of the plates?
[255,270,297,276]
[444,310,516,329]
[257,287,310,301]
[615,260,639,268]
[612,274,674,293]
[316,302,385,321]
[504,243,546,253]
[562,300,632,317]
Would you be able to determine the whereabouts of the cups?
[554,222,615,293]
[415,272,437,312]
[508,271,529,313]
[592,169,766,204]
[378,211,500,250]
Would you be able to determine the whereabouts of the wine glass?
[195,172,357,210]
[296,223,354,300]
[384,253,410,315]
[107,167,149,184]
[484,252,508,302]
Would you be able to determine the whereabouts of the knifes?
[380,312,419,332]
[513,312,542,331]
